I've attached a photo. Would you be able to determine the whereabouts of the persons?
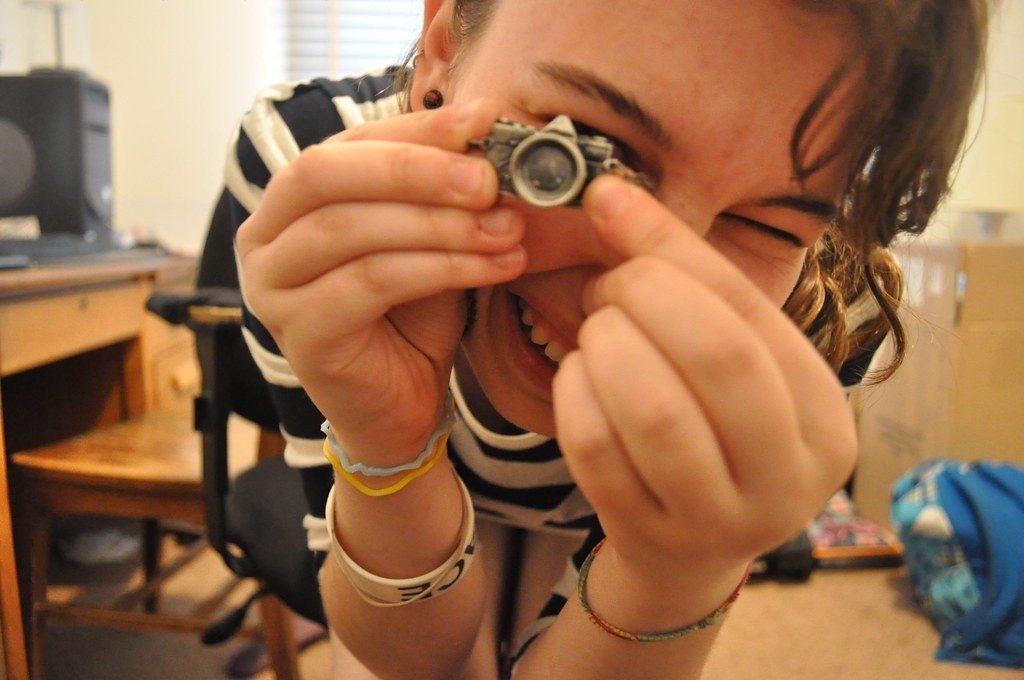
[190,0,995,680]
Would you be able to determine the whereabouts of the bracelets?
[320,388,457,479]
[324,460,478,603]
[327,458,470,609]
[575,537,754,644]
[320,395,458,497]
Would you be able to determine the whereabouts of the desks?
[0,246,268,619]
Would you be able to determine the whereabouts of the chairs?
[8,302,308,678]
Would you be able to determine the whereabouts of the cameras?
[485,118,616,214]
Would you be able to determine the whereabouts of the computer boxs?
[0,65,113,258]
